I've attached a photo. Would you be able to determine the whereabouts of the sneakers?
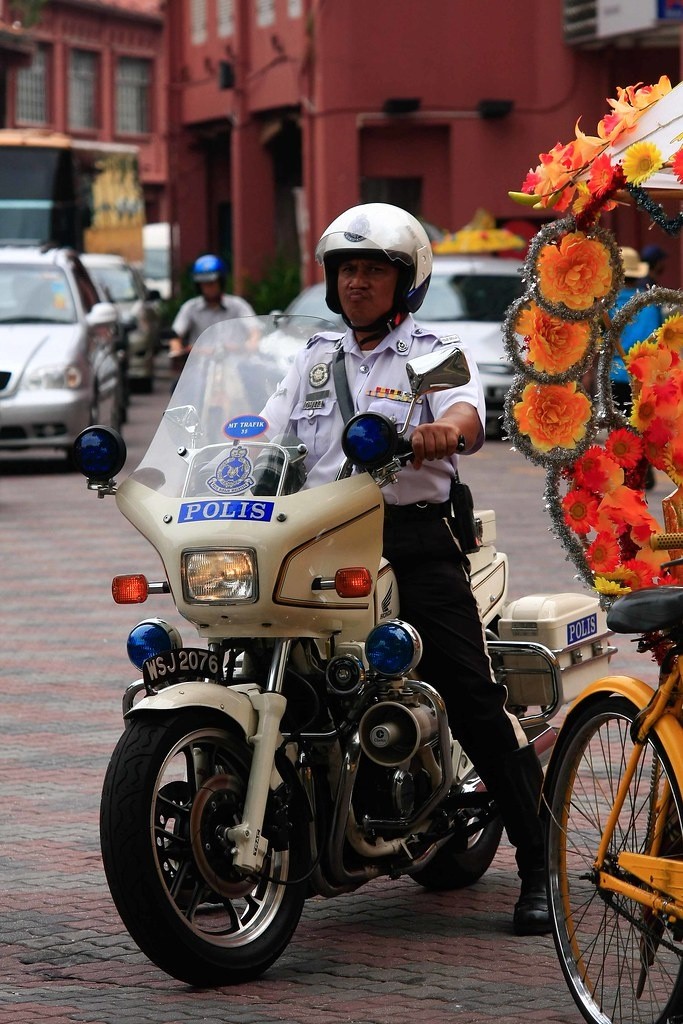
[512,866,555,931]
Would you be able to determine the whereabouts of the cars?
[25,276,138,422]
[413,260,530,439]
[256,282,345,371]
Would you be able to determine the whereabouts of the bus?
[0,128,146,263]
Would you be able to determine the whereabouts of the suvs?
[0,242,124,472]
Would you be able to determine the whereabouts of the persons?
[169,253,265,399]
[177,203,571,935]
[598,246,665,490]
[635,244,669,292]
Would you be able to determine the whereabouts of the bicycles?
[538,534,683,1024]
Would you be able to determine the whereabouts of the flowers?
[502,73,683,663]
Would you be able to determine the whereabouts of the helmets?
[316,203,432,347]
[193,254,225,282]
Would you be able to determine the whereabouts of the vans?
[44,254,163,394]
[137,222,171,300]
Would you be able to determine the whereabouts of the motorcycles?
[72,315,618,986]
